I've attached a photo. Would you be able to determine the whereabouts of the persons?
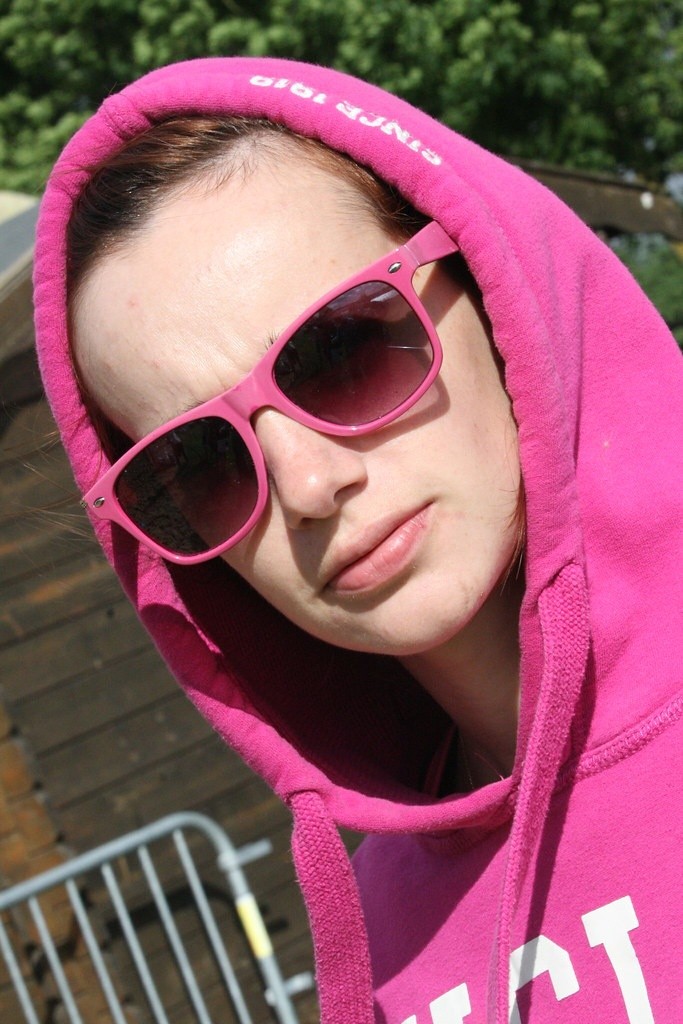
[32,57,682,1023]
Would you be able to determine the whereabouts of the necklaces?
[455,731,477,791]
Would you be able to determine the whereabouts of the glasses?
[81,220,459,566]
